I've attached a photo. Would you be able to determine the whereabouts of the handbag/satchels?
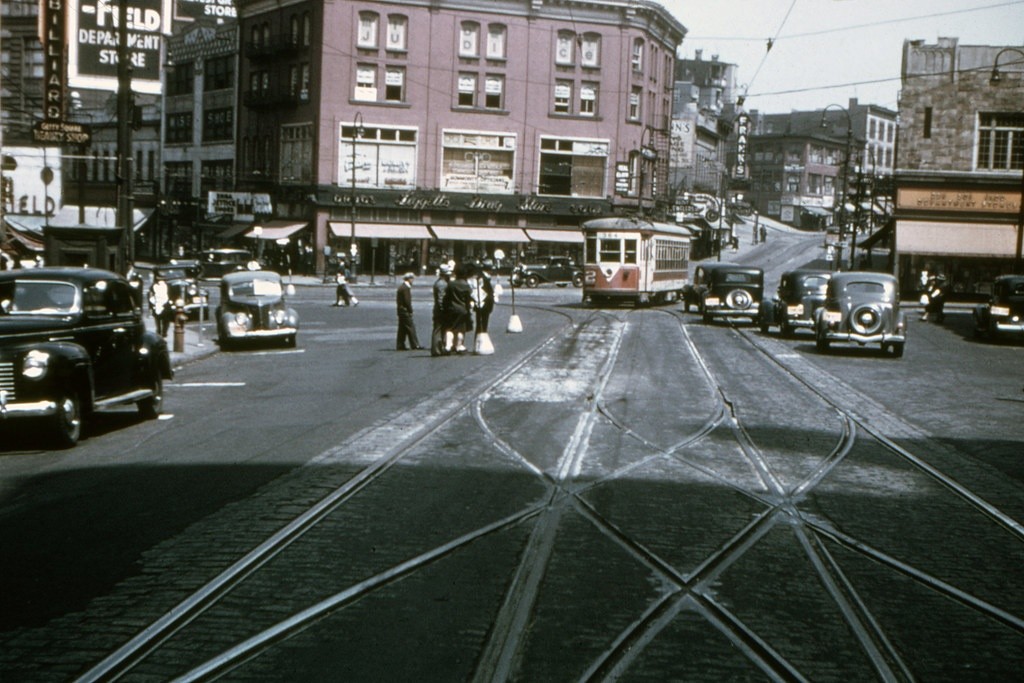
[920,294,930,305]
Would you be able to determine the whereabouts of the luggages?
[350,296,359,305]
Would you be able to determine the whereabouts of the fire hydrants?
[173,308,190,353]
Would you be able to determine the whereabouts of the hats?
[403,272,415,280]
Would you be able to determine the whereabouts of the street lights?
[819,104,856,270]
[636,125,670,217]
[850,133,875,271]
[348,111,365,282]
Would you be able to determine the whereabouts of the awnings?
[329,222,432,239]
[803,207,833,216]
[431,226,531,242]
[703,217,731,229]
[838,200,894,214]
[245,222,306,239]
[526,228,584,242]
[687,224,702,231]
[733,213,744,224]
[216,224,248,238]
[896,221,1019,257]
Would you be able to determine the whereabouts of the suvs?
[0,267,174,443]
[815,271,909,356]
[682,260,834,338]
[513,254,584,288]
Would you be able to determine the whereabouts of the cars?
[125,244,258,321]
[218,269,299,347]
[970,274,1024,340]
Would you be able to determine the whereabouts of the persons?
[333,273,349,306]
[148,276,171,337]
[396,272,424,350]
[431,255,494,355]
[918,273,946,322]
[760,225,766,242]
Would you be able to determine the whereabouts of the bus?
[580,215,691,305]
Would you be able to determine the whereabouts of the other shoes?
[919,318,927,322]
[440,350,450,356]
[433,352,440,356]
[403,347,408,350]
[413,345,424,350]
